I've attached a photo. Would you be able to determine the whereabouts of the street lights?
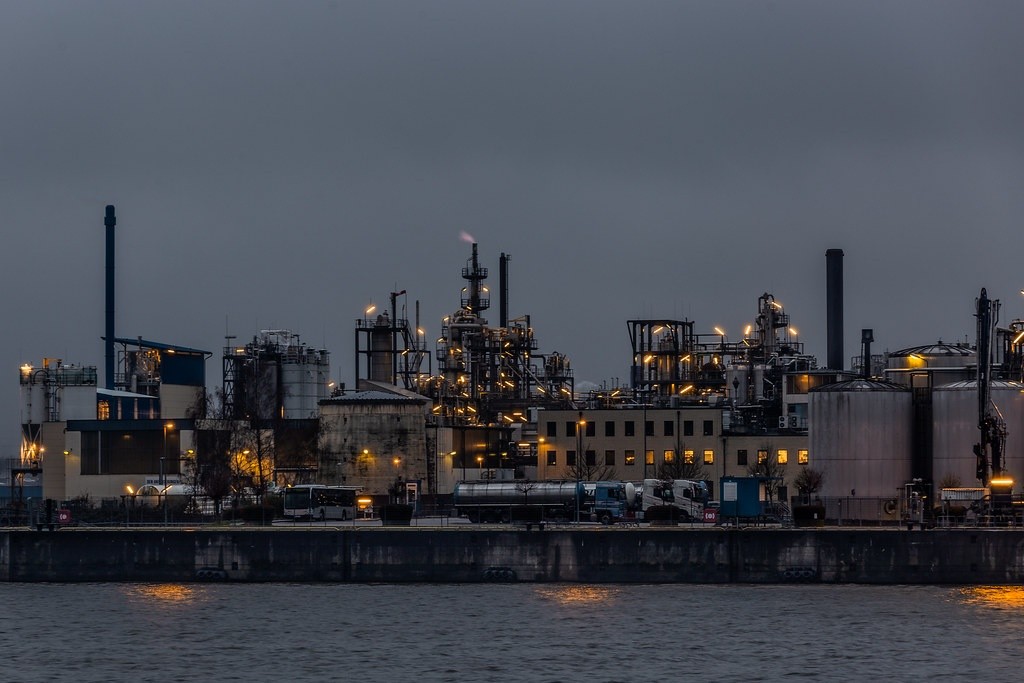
[164,422,174,528]
[477,456,483,478]
[576,419,586,479]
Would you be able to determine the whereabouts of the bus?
[284,483,357,521]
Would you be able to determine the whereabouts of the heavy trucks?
[453,478,629,526]
[582,478,677,523]
[670,478,705,525]
